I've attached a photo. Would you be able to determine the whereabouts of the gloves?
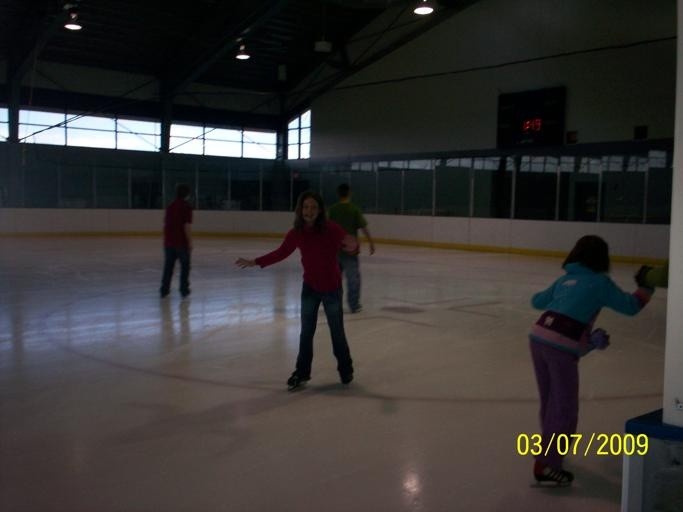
[633,264,654,289]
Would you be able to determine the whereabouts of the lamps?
[63,12,82,30]
[414,0,434,15]
[235,44,251,59]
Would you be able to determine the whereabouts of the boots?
[287,368,311,386]
[533,460,573,484]
[339,367,353,384]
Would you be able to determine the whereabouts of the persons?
[233,181,377,387]
[527,235,668,483]
[159,183,193,297]
